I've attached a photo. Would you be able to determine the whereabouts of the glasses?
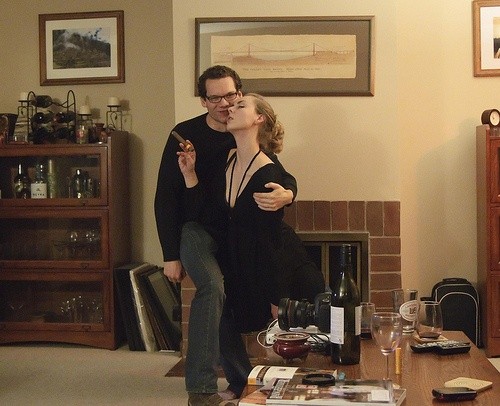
[205,90,239,103]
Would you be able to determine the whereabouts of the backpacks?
[432,278,479,347]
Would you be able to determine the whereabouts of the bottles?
[329,244,362,364]
[14,160,96,199]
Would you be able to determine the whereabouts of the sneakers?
[188,392,235,406]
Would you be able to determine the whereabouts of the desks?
[237,330,500,406]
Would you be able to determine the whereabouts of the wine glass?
[371,312,403,378]
[84,230,96,259]
[67,231,80,258]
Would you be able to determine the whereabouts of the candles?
[20,92,30,100]
[108,97,120,105]
[79,105,91,114]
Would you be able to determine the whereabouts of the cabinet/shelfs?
[476,124,500,358]
[0,131,127,351]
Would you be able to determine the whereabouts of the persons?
[153,65,297,406]
[176,92,324,400]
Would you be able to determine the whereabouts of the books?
[240,365,408,406]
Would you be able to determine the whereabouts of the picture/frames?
[195,15,375,96]
[39,10,125,86]
[472,0,500,78]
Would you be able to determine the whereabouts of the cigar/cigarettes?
[171,131,194,152]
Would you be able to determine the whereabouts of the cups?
[417,301,443,341]
[75,124,88,144]
[392,288,418,335]
[360,302,377,340]
